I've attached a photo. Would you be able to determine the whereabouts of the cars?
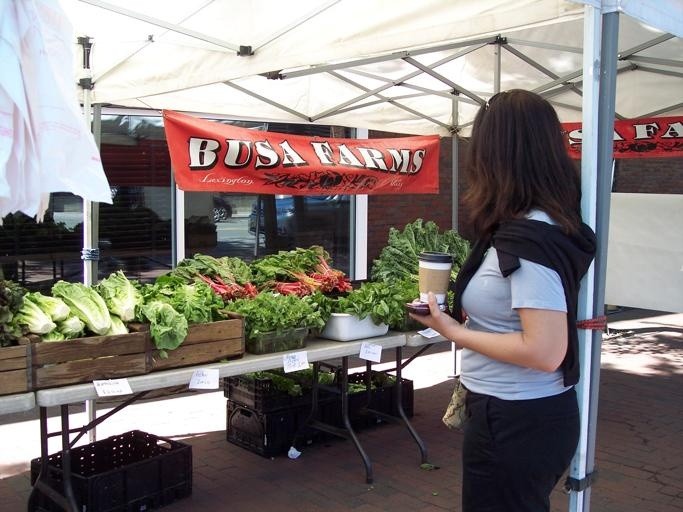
[110,185,232,222]
[248,195,349,244]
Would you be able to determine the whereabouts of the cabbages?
[5,270,142,343]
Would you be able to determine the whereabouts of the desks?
[353,324,447,469]
[26,329,407,510]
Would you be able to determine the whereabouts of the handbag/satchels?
[440,378,469,433]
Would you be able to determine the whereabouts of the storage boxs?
[29,332,147,390]
[129,312,246,369]
[0,339,33,397]
[246,329,308,354]
[325,310,389,341]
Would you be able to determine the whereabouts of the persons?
[408,85,601,512]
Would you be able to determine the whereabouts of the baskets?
[29,429,194,512]
[222,360,417,459]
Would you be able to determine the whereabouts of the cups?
[417,250,453,305]
